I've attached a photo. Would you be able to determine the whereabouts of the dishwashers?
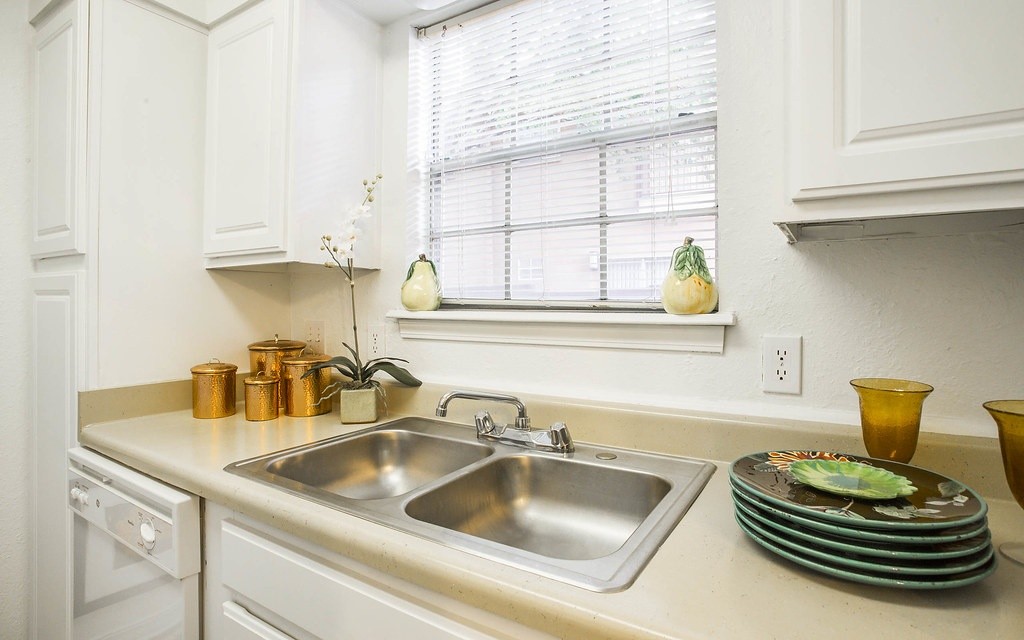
[66,447,203,640]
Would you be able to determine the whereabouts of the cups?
[850,378,934,464]
[281,348,332,416]
[189,358,237,419]
[242,371,280,421]
[248,333,307,408]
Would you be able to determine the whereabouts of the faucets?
[435,390,531,431]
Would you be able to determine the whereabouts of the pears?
[400,253,443,311]
[661,237,718,314]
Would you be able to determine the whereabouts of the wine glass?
[983,400,1024,564]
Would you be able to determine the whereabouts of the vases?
[340,383,380,423]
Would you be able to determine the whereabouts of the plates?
[727,450,997,589]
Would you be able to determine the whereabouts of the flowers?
[302,175,423,389]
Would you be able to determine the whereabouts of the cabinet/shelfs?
[26,0,209,640]
[204,500,563,640]
[204,0,382,274]
[773,0,1024,243]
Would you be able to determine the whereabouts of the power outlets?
[366,325,387,362]
[762,338,802,394]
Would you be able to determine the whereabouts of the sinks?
[403,454,716,594]
[223,417,495,501]
